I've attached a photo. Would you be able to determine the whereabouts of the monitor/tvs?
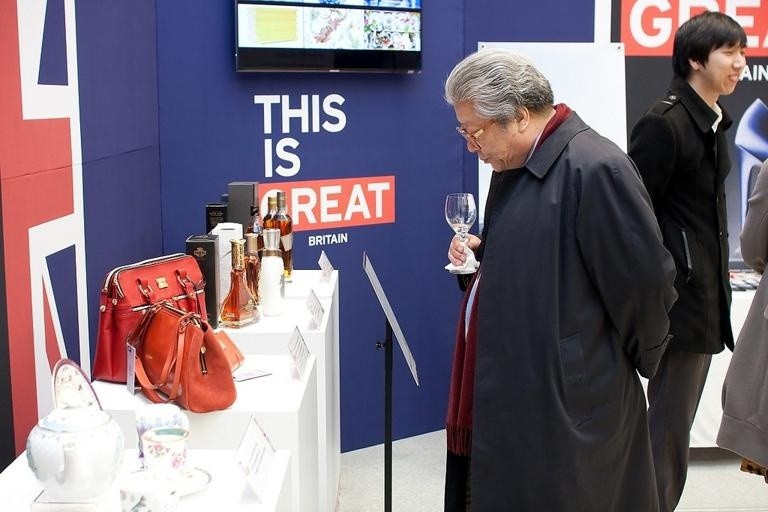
[232,0,424,75]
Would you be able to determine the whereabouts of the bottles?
[219,190,294,329]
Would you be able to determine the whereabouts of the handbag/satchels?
[91,253,237,412]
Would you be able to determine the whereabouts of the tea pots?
[25,408,126,502]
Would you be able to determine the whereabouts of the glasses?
[456,119,495,151]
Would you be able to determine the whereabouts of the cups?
[142,425,191,485]
[135,403,192,457]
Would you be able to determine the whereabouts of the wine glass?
[444,192,477,275]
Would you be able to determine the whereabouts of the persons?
[445,47,679,512]
[715,155,768,484]
[628,12,749,512]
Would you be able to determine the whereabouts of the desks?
[1,262,345,512]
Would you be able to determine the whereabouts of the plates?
[50,358,107,411]
[120,463,213,503]
